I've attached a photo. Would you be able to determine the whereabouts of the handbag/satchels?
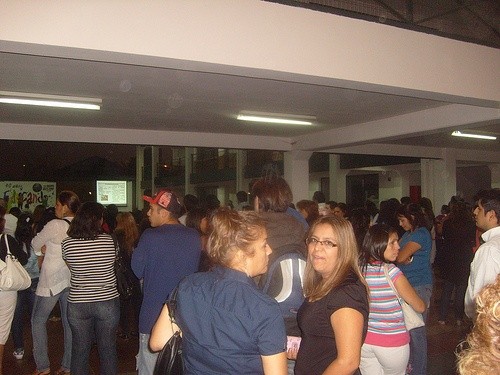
[152,287,182,375]
[383,263,426,331]
[110,233,139,300]
[0,233,33,292]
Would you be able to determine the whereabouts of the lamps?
[450,129,500,141]
[236,110,317,126]
[0,91,102,110]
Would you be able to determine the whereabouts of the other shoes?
[437,320,446,325]
[13,348,25,360]
[456,320,464,325]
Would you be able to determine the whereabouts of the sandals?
[31,368,52,375]
[53,368,71,375]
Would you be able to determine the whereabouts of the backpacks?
[258,243,319,337]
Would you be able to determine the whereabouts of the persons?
[0,174,500,375]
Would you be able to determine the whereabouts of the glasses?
[305,238,337,249]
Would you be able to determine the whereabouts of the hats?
[143,190,181,212]
